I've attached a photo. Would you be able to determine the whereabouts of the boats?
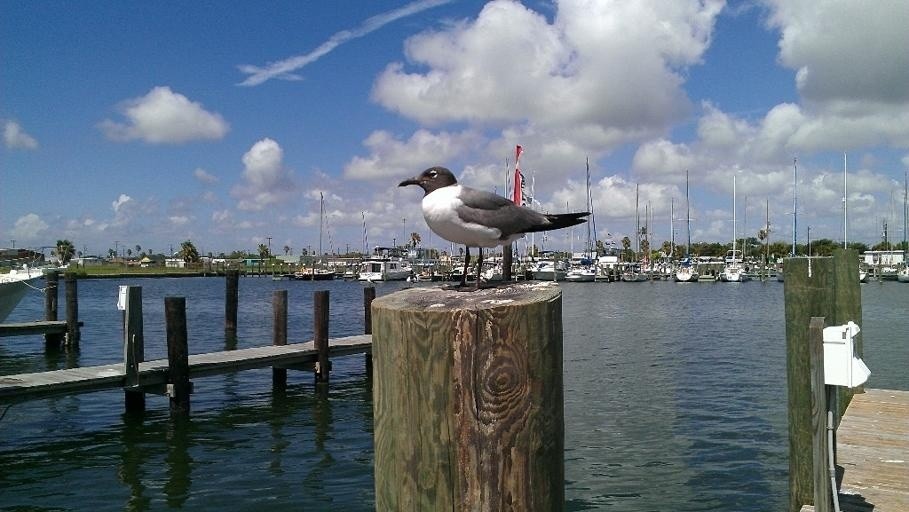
[358,255,413,283]
[10,262,68,279]
[0,246,47,325]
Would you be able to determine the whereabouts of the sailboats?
[840,151,867,283]
[881,189,897,277]
[293,191,337,281]
[896,170,909,282]
[414,153,814,281]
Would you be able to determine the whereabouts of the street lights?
[288,262,291,274]
[280,263,283,274]
[272,263,275,277]
[302,262,305,269]
[295,262,298,270]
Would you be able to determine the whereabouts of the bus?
[326,257,362,270]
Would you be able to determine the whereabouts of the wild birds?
[397,166,592,293]
